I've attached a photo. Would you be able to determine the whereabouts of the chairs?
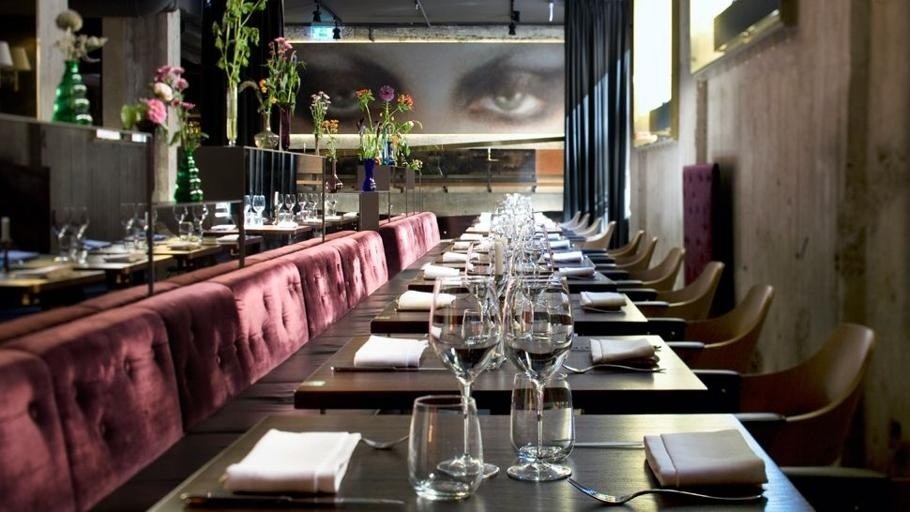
[643,283,775,370]
[210,259,310,384]
[127,280,260,437]
[692,322,877,467]
[2,347,77,511]
[296,209,774,338]
[780,467,908,510]
[3,304,186,510]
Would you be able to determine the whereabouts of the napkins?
[355,334,429,370]
[589,336,659,363]
[222,426,364,495]
[643,429,770,491]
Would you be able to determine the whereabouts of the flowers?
[353,85,413,160]
[238,37,294,114]
[145,65,210,149]
[309,91,331,155]
[323,119,339,161]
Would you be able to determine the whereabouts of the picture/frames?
[631,0,680,149]
[685,0,797,77]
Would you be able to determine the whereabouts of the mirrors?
[149,199,243,300]
[1,117,153,342]
[241,148,326,268]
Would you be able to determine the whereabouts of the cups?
[411,393,482,503]
[509,372,576,464]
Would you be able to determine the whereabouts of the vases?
[361,158,377,191]
[174,145,204,203]
[254,109,280,149]
[382,139,396,166]
[324,156,344,193]
[314,131,320,155]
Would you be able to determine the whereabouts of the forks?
[361,427,422,449]
[565,476,767,504]
[562,362,665,375]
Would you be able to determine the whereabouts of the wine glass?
[56,192,337,267]
[428,273,501,479]
[470,193,555,370]
[503,282,573,484]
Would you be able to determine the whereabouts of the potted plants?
[260,60,307,149]
[212,0,267,148]
[54,9,109,125]
[396,139,411,166]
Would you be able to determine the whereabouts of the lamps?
[0,40,14,68]
[309,0,345,42]
[11,47,31,93]
[507,1,522,36]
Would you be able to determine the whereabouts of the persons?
[288,43,565,134]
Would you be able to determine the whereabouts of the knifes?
[330,364,450,372]
[179,485,406,505]
[556,441,643,447]
[573,345,661,353]
